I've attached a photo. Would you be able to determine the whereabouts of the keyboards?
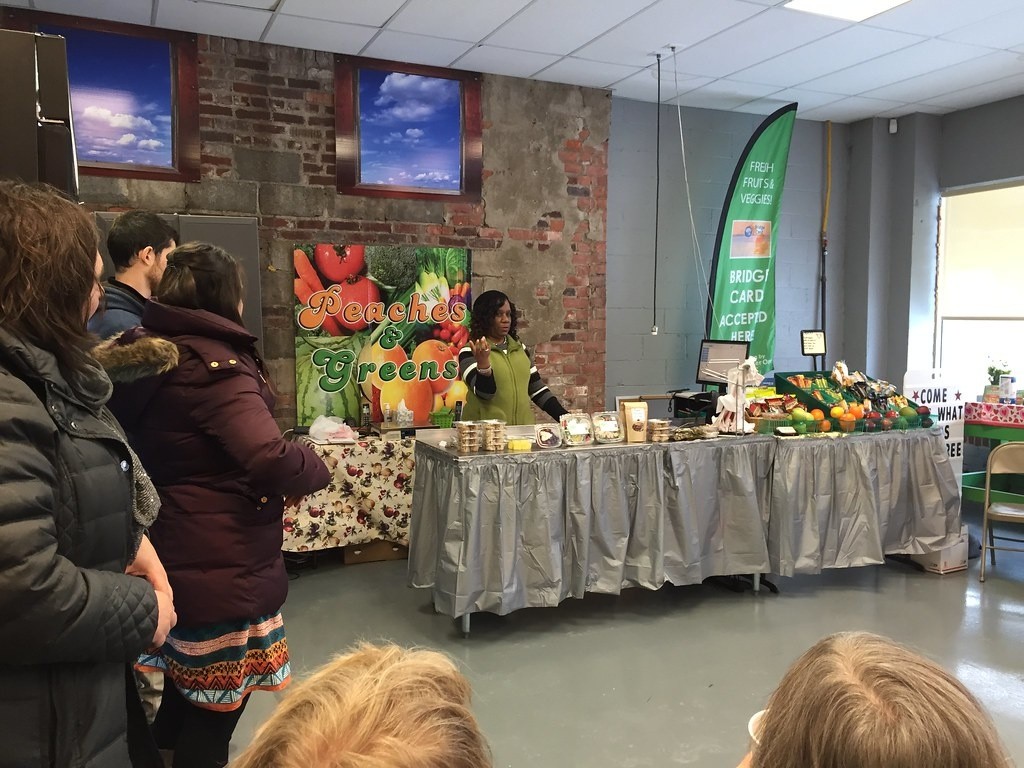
[695,393,715,401]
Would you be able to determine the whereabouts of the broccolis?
[363,245,416,290]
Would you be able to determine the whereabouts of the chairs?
[980,441,1024,581]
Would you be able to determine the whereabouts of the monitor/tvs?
[696,339,750,394]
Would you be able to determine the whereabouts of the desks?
[281,433,416,568]
[415,424,960,636]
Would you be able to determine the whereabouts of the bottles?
[362,404,370,426]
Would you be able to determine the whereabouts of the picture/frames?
[333,53,483,208]
[2,4,201,185]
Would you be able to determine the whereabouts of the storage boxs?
[774,369,921,433]
[909,522,969,575]
[338,539,409,565]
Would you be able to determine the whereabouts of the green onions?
[370,285,425,350]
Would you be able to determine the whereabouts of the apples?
[864,411,900,432]
[915,406,932,427]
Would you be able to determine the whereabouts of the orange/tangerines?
[370,337,457,425]
[811,405,865,432]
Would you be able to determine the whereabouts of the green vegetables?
[414,247,467,290]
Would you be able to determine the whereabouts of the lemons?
[433,381,470,412]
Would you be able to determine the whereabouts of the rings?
[485,349,489,351]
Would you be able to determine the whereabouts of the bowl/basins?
[592,410,624,444]
[536,425,562,448]
[455,418,506,453]
[561,413,594,446]
[648,418,718,441]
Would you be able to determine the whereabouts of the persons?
[87,243,338,768]
[88,210,183,347]
[0,178,178,768]
[458,290,571,426]
[735,630,1017,768]
[223,639,491,768]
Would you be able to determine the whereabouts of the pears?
[788,407,815,433]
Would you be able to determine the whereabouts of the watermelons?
[292,313,371,429]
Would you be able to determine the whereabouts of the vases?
[982,385,1000,404]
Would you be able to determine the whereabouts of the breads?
[786,361,908,410]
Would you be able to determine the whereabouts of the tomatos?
[432,318,456,342]
[315,245,383,330]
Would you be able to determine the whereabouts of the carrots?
[451,326,470,349]
[293,249,341,337]
[450,282,470,297]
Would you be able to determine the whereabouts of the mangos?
[894,406,919,430]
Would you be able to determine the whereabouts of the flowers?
[984,353,1012,384]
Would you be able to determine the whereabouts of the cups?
[748,709,769,759]
